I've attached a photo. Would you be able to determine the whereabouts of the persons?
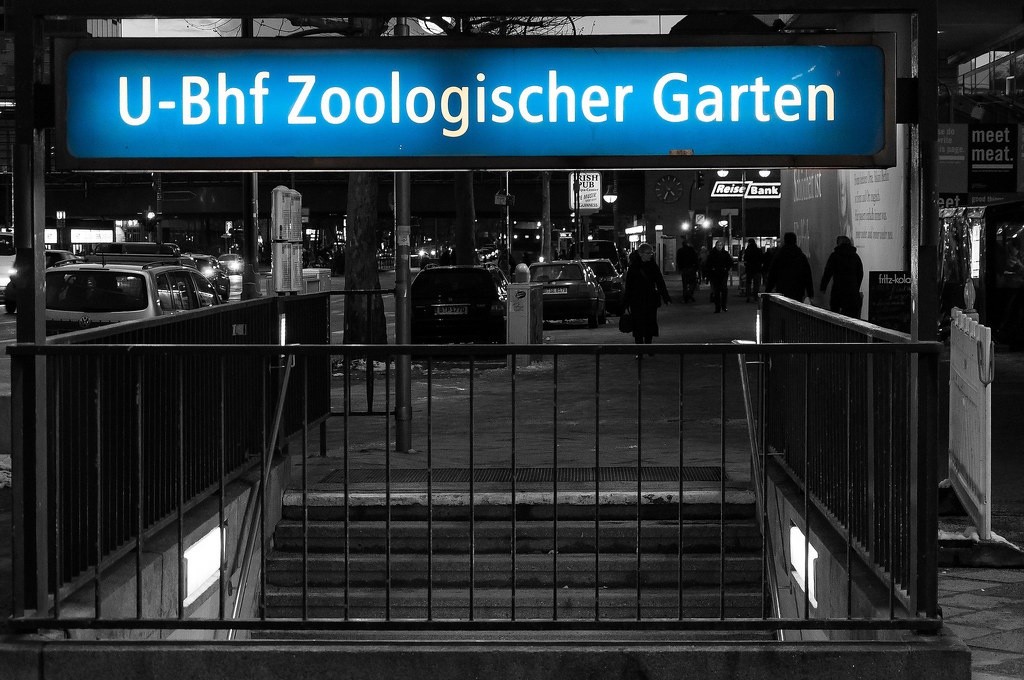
[624,243,673,360]
[700,248,710,284]
[677,240,699,304]
[765,232,815,304]
[702,242,734,313]
[744,238,763,302]
[820,236,863,316]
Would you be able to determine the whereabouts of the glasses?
[643,252,654,256]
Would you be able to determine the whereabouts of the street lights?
[716,169,770,249]
[56,210,65,251]
[573,169,618,261]
[146,206,156,241]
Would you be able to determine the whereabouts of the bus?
[85,242,181,267]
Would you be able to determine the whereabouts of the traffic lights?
[696,170,704,191]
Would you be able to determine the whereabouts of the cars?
[186,253,230,301]
[217,253,244,275]
[580,259,625,317]
[529,260,607,328]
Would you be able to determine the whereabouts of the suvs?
[410,263,510,361]
[43,258,228,340]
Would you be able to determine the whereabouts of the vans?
[569,240,623,276]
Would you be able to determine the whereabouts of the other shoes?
[635,354,656,359]
[714,306,729,314]
